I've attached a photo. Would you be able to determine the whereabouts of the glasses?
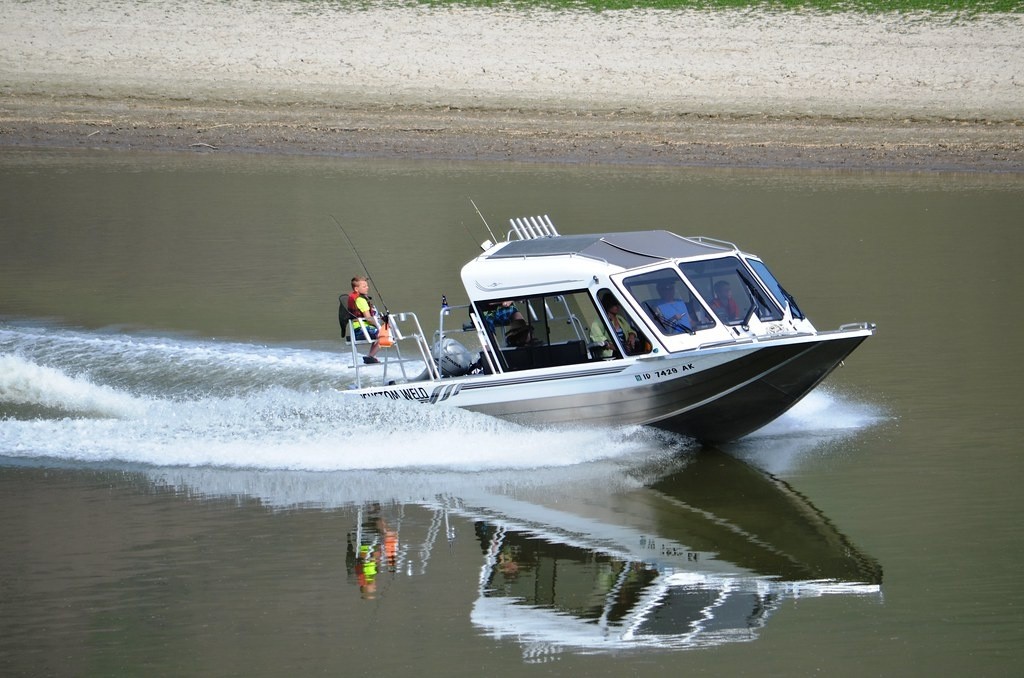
[661,286,674,290]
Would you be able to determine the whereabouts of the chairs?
[657,301,697,335]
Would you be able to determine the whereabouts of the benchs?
[348,311,438,382]
[437,294,588,381]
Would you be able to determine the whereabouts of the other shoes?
[362,354,381,364]
[526,337,544,346]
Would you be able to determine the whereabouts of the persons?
[347,276,381,363]
[708,279,739,323]
[650,278,697,335]
[589,292,645,360]
[467,298,530,347]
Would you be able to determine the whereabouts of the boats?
[354,443,882,652]
[338,199,875,444]
